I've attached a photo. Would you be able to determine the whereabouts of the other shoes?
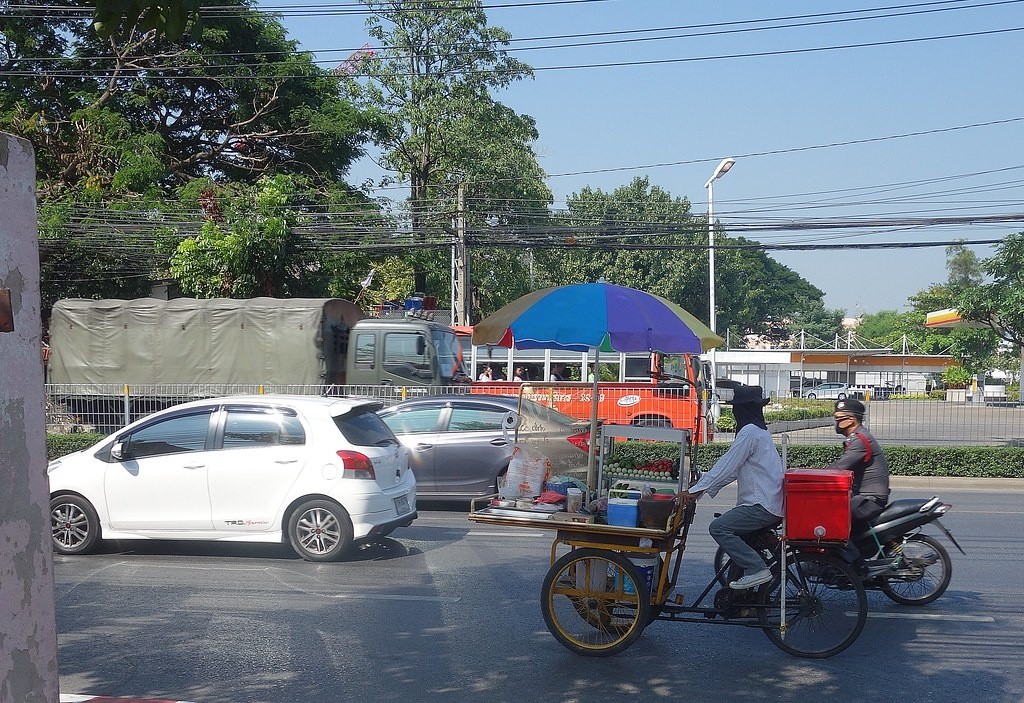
[837,566,871,587]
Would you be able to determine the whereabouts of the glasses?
[834,415,851,419]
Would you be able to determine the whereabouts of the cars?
[790,379,849,401]
[375,395,610,507]
[46,394,418,562]
[707,378,749,403]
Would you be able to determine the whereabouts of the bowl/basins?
[637,500,675,528]
[491,499,502,505]
[500,501,515,507]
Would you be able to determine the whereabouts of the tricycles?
[468,381,868,658]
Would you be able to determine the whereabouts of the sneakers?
[729,568,773,590]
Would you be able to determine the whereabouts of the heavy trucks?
[46,298,472,423]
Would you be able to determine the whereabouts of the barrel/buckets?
[614,553,657,598]
[575,559,607,592]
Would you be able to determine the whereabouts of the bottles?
[640,484,653,499]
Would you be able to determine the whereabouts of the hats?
[834,399,865,415]
[725,386,770,406]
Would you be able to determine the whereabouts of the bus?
[452,326,715,445]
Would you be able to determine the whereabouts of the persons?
[668,386,785,590]
[480,363,600,382]
[825,399,891,578]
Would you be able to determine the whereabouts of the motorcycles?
[715,494,965,606]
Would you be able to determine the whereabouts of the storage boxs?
[638,496,675,530]
[608,489,642,528]
[785,468,854,540]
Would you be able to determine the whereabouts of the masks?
[835,418,855,436]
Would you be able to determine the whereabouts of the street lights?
[705,158,736,422]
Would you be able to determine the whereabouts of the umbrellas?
[474,283,726,488]
